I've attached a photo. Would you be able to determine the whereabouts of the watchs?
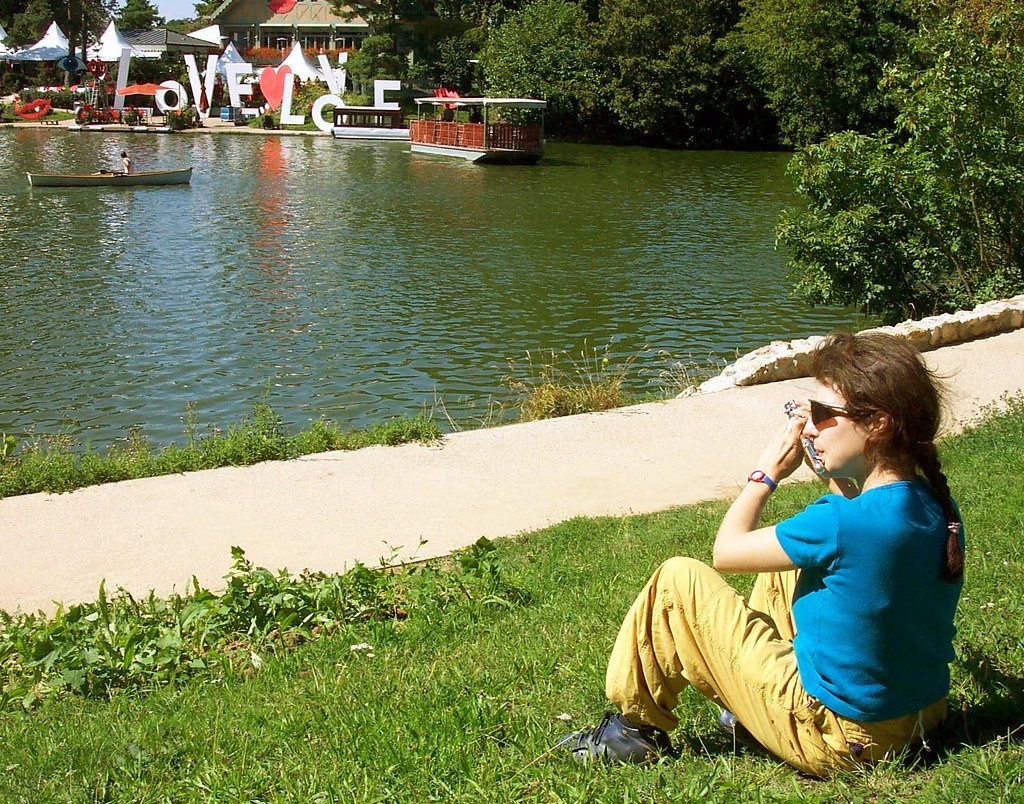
[748,468,779,494]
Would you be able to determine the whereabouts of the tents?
[58,19,148,81]
[240,38,329,84]
[200,39,264,86]
[1,24,29,69]
[12,21,85,78]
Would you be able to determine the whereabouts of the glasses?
[807,398,875,427]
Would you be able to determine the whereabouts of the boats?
[406,96,548,163]
[331,105,411,141]
[26,166,193,188]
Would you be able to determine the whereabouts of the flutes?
[783,399,827,475]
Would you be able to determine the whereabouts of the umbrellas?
[143,82,175,109]
[116,83,158,109]
[199,81,211,127]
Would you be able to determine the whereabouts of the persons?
[553,326,970,779]
[472,106,482,124]
[478,107,485,123]
[120,150,136,174]
[444,102,455,122]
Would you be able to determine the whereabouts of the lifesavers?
[154,80,188,116]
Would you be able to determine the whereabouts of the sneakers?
[555,710,672,771]
[718,709,768,752]
